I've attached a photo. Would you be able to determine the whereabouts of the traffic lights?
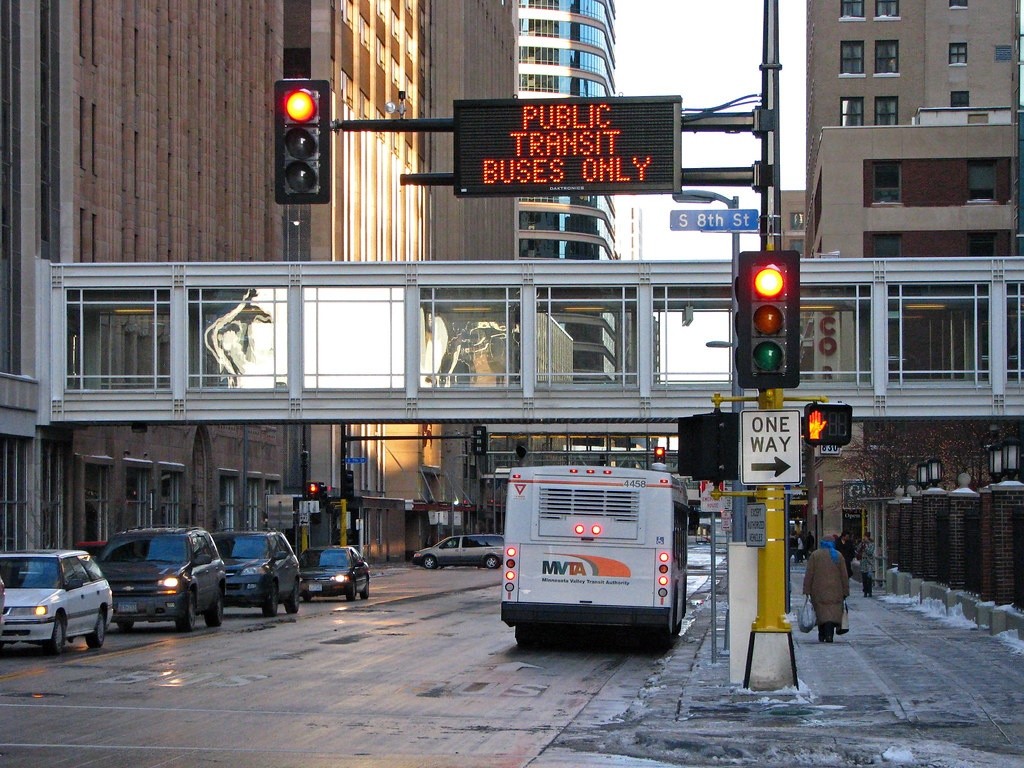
[307,481,321,496]
[273,77,331,205]
[803,403,853,446]
[738,249,800,388]
[319,482,329,502]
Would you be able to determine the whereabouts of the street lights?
[672,189,746,651]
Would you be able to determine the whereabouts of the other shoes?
[869,594,872,597]
[826,635,833,642]
[819,636,824,642]
[864,594,867,597]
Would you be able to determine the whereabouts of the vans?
[413,533,504,570]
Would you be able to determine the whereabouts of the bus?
[500,466,700,645]
[0,549,113,652]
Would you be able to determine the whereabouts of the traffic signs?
[740,409,802,485]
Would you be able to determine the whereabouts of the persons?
[855,532,875,597]
[802,533,850,643]
[833,529,862,578]
[788,516,815,563]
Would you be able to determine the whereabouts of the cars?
[297,545,370,601]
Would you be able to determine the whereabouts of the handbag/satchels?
[797,594,817,633]
[836,599,849,635]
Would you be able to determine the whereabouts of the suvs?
[209,527,300,617]
[95,524,226,632]
